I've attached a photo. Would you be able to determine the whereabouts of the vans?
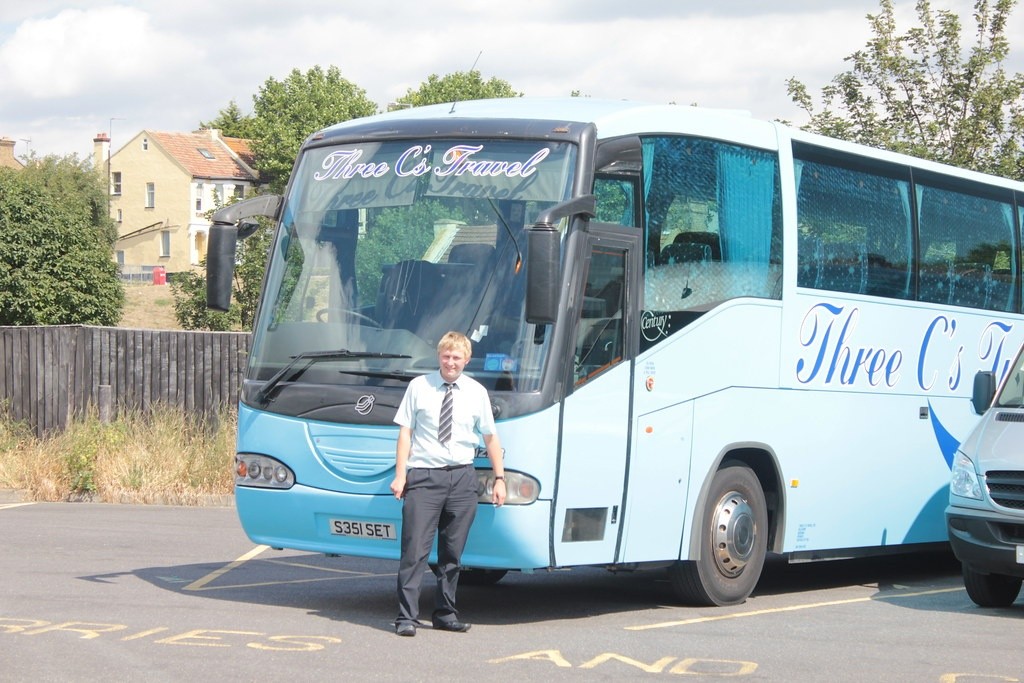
[944,343,1024,604]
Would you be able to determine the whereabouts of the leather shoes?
[396,623,416,636]
[433,619,472,632]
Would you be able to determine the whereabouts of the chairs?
[447,242,496,270]
[376,258,441,337]
[654,228,1024,314]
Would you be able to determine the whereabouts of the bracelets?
[496,476,504,480]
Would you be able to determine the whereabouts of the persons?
[390,331,506,636]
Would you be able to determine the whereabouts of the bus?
[206,89,1023,606]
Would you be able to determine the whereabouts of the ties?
[439,384,456,449]
